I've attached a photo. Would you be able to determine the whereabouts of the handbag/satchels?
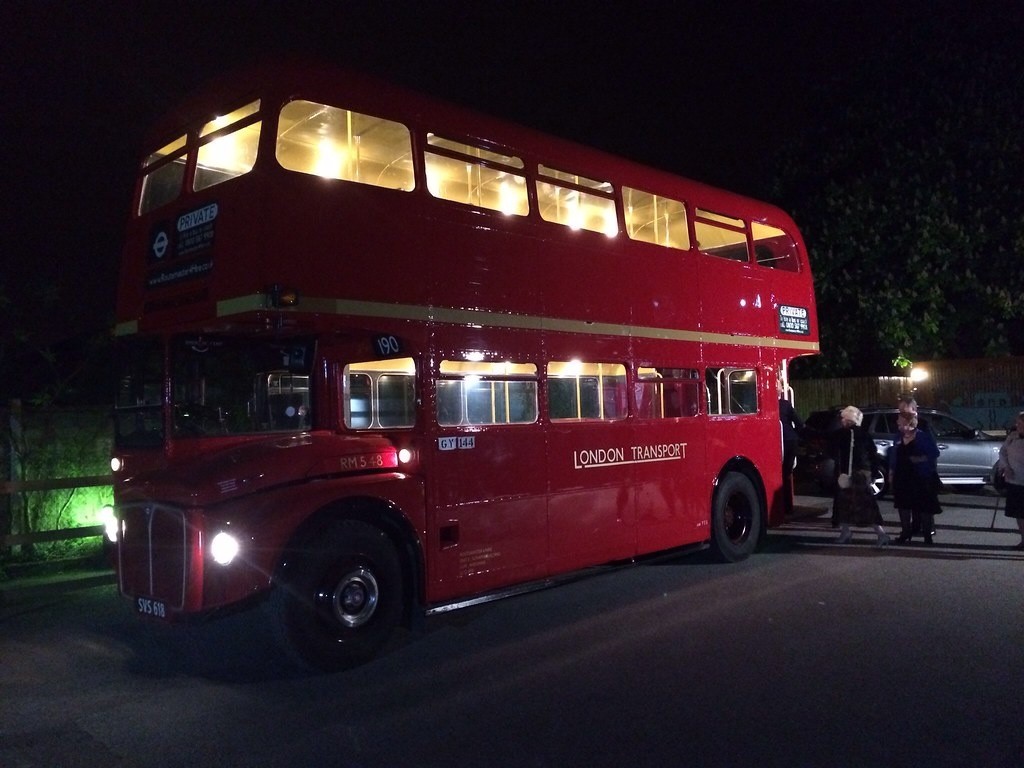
[989,461,1006,492]
[836,470,869,491]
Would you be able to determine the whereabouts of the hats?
[841,406,864,427]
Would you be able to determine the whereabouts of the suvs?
[798,403,1008,500]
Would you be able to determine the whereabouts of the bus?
[109,59,821,679]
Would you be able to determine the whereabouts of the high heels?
[871,533,891,549]
[832,530,852,544]
[924,532,933,545]
[894,531,914,543]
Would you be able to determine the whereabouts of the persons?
[997,412,1024,550]
[892,396,943,544]
[776,379,891,547]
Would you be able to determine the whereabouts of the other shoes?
[1014,543,1024,551]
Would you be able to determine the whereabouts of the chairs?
[276,391,306,430]
